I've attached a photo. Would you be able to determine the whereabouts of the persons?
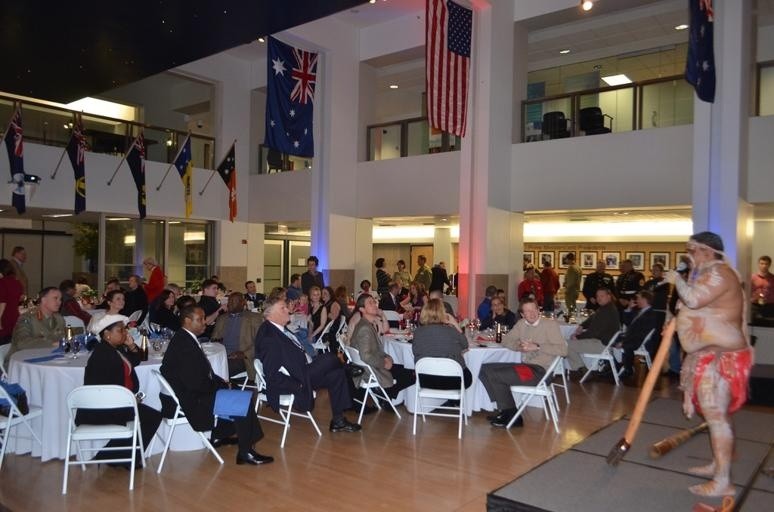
[2,247,690,470]
[752,255,774,327]
[663,232,754,497]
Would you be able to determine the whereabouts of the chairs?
[252,359,324,448]
[147,369,224,475]
[553,358,571,411]
[61,385,146,494]
[342,347,403,424]
[0,368,44,467]
[633,328,653,370]
[539,110,571,140]
[576,107,612,137]
[504,355,561,434]
[579,331,623,387]
[2,295,407,392]
[412,358,468,440]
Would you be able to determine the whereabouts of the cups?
[501,324,509,334]
[487,329,495,341]
[543,303,588,323]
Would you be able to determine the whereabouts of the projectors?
[13,170,44,186]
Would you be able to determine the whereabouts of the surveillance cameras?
[197,119,204,128]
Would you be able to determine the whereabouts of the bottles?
[191,285,199,294]
[495,322,501,343]
[349,292,354,303]
[22,293,39,309]
[404,326,410,336]
[258,301,263,313]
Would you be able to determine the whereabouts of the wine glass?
[468,318,481,335]
[59,324,171,361]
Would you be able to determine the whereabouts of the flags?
[173,137,196,218]
[4,106,26,214]
[125,131,146,220]
[425,0,472,139]
[263,34,319,159]
[66,113,87,217]
[684,0,716,103]
[217,144,237,224]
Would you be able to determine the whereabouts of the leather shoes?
[211,433,238,447]
[345,364,363,378]
[330,417,361,433]
[487,408,522,427]
[237,449,273,464]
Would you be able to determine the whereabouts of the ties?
[253,296,255,300]
[284,326,303,349]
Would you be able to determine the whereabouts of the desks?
[544,311,588,376]
[4,339,229,466]
[380,330,521,417]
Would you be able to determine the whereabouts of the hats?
[92,315,129,336]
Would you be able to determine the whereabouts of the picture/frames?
[522,251,691,293]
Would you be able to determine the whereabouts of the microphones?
[654,261,687,293]
